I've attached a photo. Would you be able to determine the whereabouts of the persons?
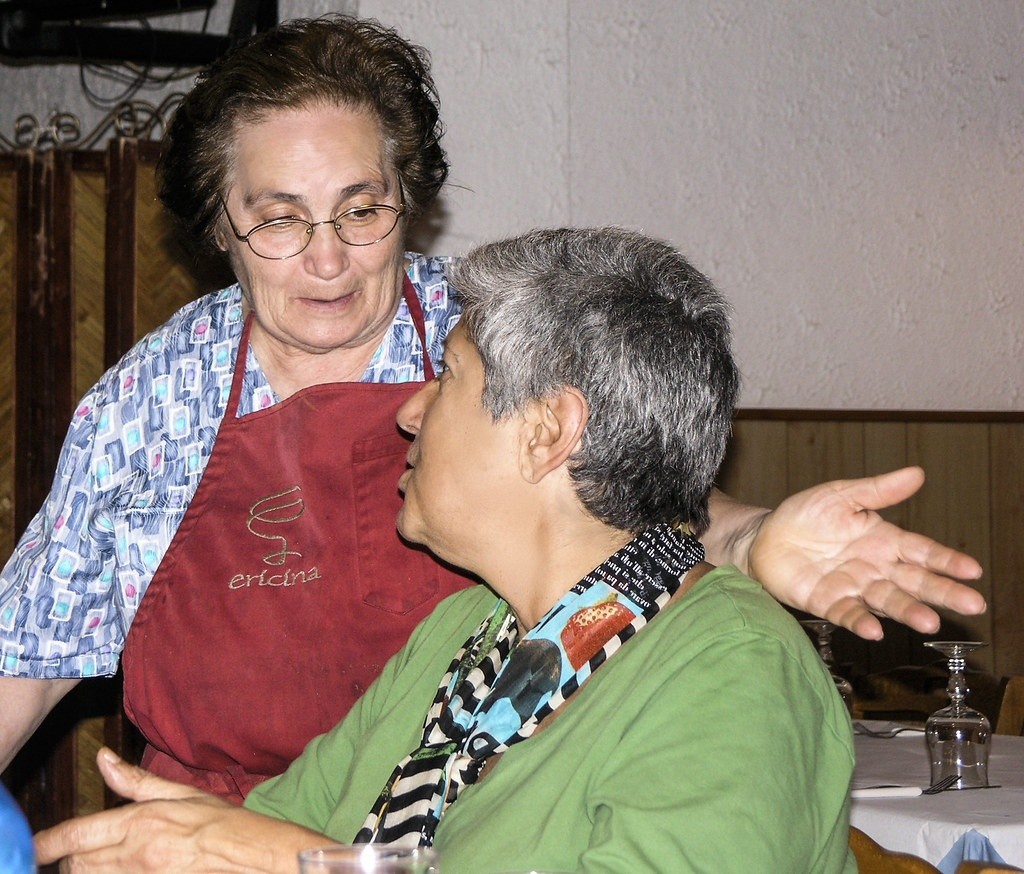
[33,227,856,874]
[0,11,988,874]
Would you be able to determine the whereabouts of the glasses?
[218,171,406,260]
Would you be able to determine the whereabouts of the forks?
[850,774,962,797]
[853,721,928,740]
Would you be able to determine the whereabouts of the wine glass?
[920,638,994,791]
[798,619,852,718]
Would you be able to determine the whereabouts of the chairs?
[839,660,1024,736]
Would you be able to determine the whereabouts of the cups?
[297,842,440,874]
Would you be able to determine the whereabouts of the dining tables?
[846,718,1024,874]
